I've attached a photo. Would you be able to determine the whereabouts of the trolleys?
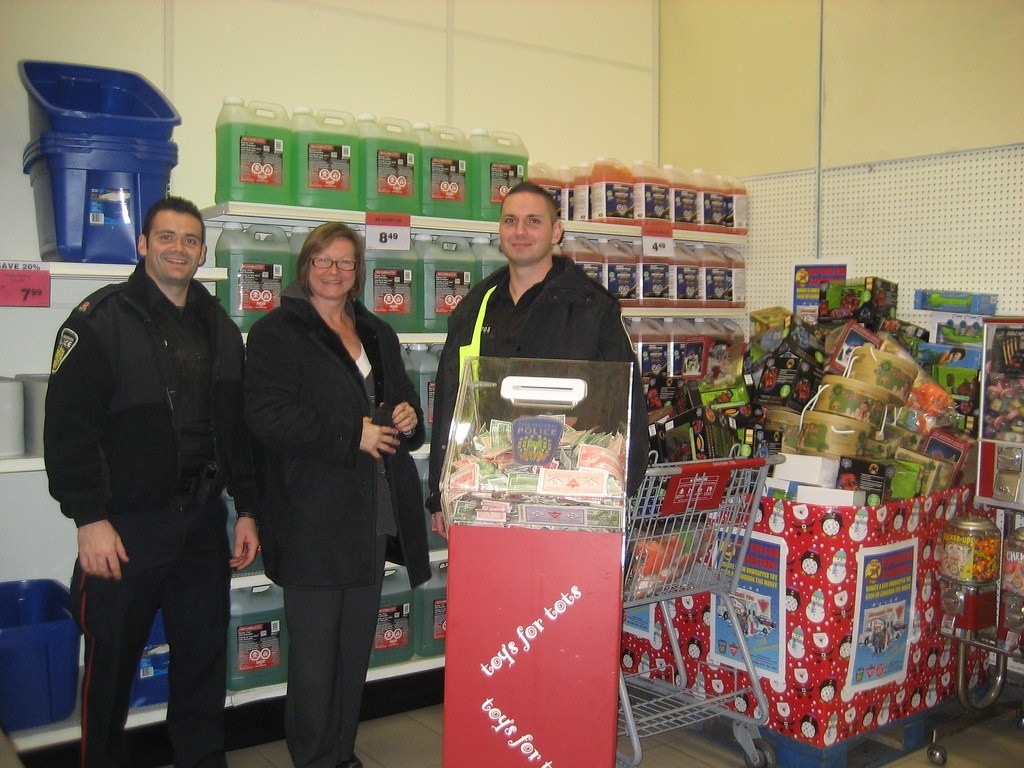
[615,443,787,768]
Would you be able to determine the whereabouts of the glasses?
[308,257,358,271]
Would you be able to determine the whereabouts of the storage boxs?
[127,608,170,708]
[621,482,980,746]
[0,579,79,732]
[16,60,181,264]
[637,258,999,506]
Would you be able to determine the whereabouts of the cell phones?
[371,402,398,455]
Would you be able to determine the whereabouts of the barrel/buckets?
[367,566,414,667]
[0,578,81,732]
[222,490,265,573]
[398,344,445,445]
[419,472,448,553]
[528,158,749,382]
[411,232,476,331]
[214,96,290,206]
[289,227,312,285]
[17,58,180,267]
[413,122,481,219]
[127,609,168,708]
[358,114,423,215]
[227,582,290,692]
[414,560,448,657]
[216,223,292,332]
[468,128,529,222]
[470,237,508,289]
[290,105,359,211]
[360,231,419,333]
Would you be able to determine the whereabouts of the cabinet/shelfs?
[956,319,1024,675]
[0,200,750,768]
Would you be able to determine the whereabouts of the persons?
[425,183,649,539]
[44,197,259,768]
[240,222,432,767]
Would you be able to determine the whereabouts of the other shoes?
[336,754,364,768]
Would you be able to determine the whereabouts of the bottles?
[938,516,1023,655]
